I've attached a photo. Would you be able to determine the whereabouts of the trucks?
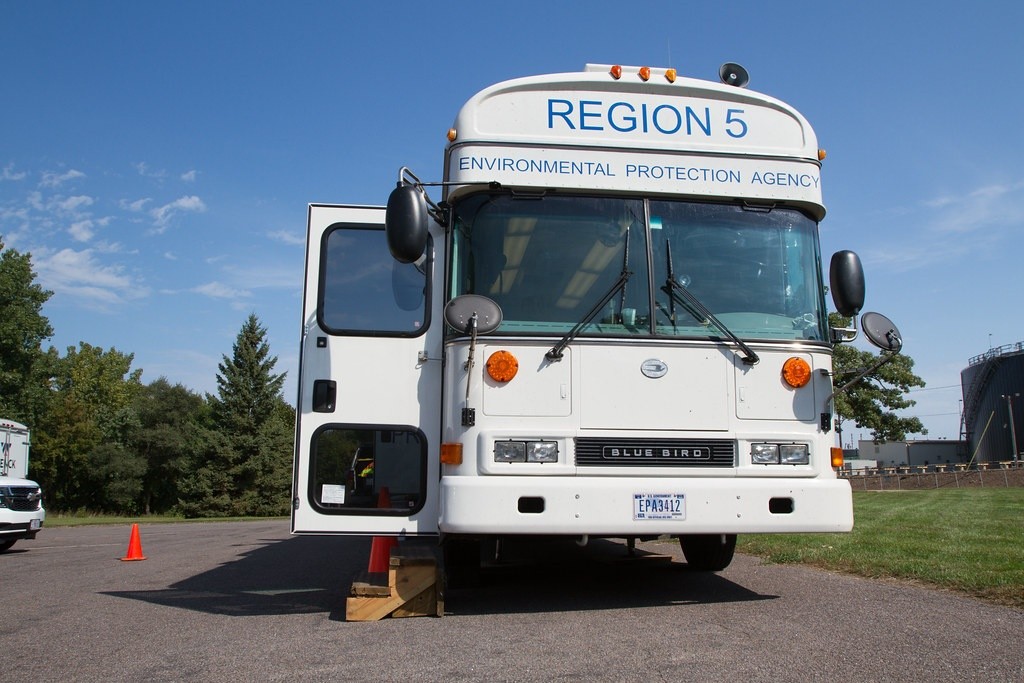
[0,418,30,480]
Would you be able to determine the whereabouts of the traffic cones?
[356,484,401,582]
[120,524,148,561]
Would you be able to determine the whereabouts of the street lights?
[1000,393,1020,468]
[959,399,963,419]
[989,333,992,357]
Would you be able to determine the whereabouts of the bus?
[289,61,905,573]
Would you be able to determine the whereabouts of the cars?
[0,475,46,553]
